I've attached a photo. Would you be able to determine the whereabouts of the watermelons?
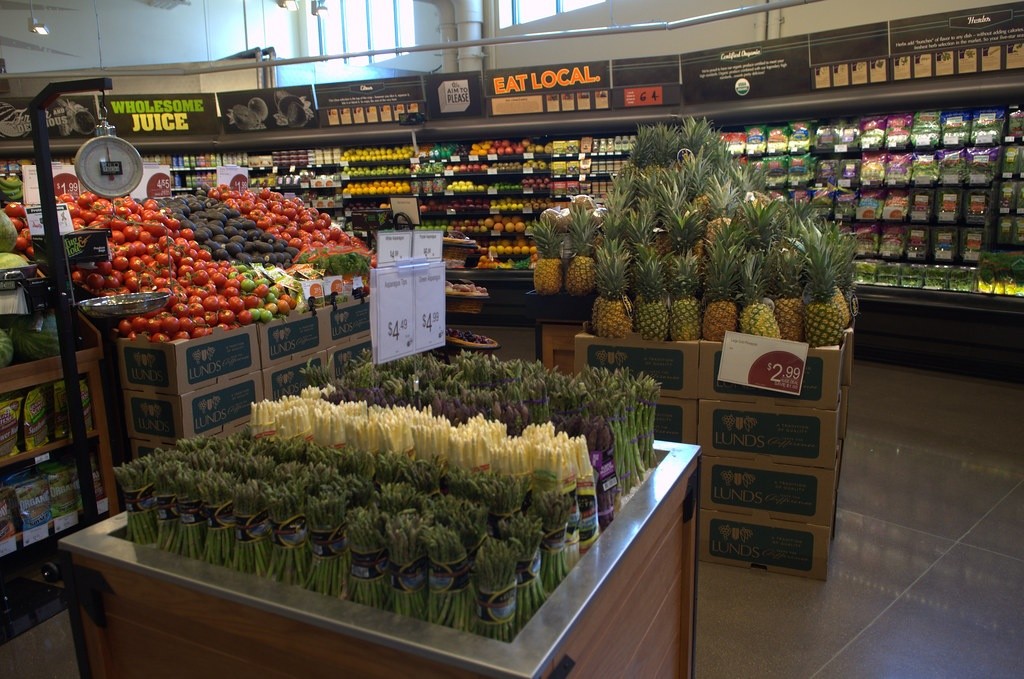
[0,210,61,369]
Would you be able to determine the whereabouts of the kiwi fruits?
[491,161,523,171]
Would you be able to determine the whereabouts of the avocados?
[149,189,301,270]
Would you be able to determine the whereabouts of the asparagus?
[113,351,662,647]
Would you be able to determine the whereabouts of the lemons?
[489,182,523,190]
[340,146,417,161]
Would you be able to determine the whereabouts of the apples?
[418,197,555,232]
[343,164,411,177]
[521,174,553,189]
[446,180,485,193]
[488,138,552,155]
[446,163,489,173]
[523,159,547,170]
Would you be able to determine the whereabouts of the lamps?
[276,0,328,16]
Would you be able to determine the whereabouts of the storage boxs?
[115,292,375,462]
[571,317,857,582]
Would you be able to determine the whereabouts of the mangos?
[470,140,493,156]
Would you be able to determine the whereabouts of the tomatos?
[358,239,376,268]
[3,190,230,299]
[206,184,362,250]
[118,265,296,342]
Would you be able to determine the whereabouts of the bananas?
[0,175,25,199]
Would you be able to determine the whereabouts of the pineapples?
[528,116,856,347]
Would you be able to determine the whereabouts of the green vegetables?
[298,249,372,274]
[814,110,1024,297]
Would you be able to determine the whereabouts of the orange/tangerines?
[476,213,538,268]
[342,179,411,195]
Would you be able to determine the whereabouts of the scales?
[64,107,172,315]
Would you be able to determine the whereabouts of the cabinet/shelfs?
[170,150,632,329]
[0,312,121,648]
